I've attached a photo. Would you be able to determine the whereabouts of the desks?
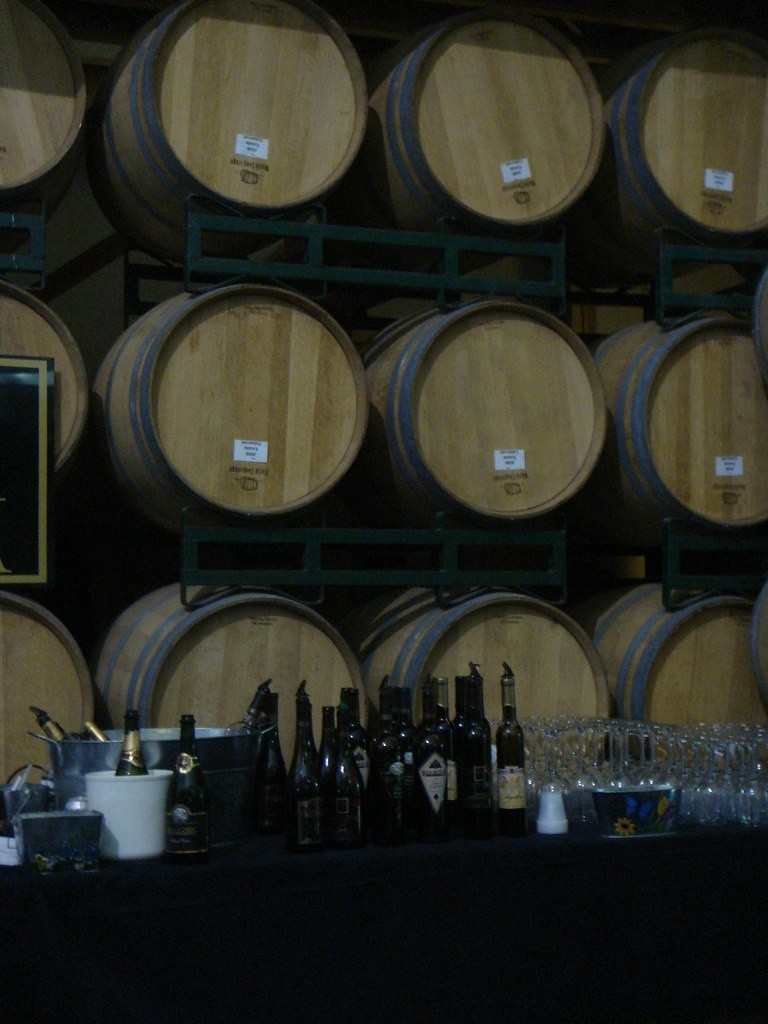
[0,799,768,1024]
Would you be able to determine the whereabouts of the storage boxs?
[11,810,103,865]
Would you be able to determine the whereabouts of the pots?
[24,721,257,849]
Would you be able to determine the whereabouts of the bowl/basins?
[594,785,681,835]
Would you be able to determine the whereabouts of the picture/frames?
[0,355,55,588]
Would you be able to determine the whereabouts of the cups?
[83,770,174,863]
[10,811,103,876]
[0,781,51,829]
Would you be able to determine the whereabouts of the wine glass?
[489,715,768,830]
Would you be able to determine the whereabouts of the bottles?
[28,705,109,740]
[536,783,568,835]
[247,694,287,835]
[237,678,272,730]
[497,662,527,837]
[114,710,149,776]
[318,662,492,849]
[164,715,208,854]
[287,679,323,845]
